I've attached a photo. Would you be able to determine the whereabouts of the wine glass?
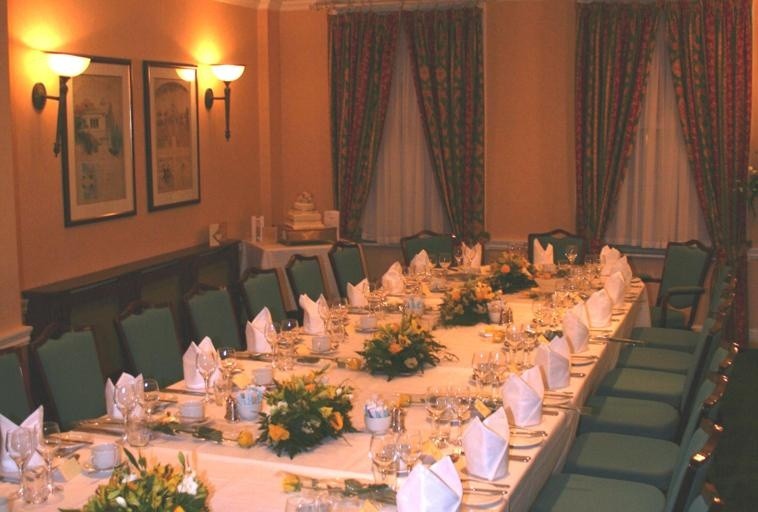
[164,236,638,405]
[112,378,161,446]
[284,384,559,511]
[4,425,34,498]
[32,420,65,498]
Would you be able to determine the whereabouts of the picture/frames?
[142,59,202,212]
[61,54,137,228]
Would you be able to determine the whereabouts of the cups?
[89,441,123,470]
[235,388,265,421]
[20,464,49,508]
[172,401,206,421]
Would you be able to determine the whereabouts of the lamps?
[32,52,91,160]
[205,63,247,142]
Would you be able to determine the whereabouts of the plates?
[171,415,213,426]
[48,430,93,453]
[90,414,125,435]
[80,456,128,473]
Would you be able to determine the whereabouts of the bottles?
[223,396,239,423]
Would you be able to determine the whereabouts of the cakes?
[285,191,324,230]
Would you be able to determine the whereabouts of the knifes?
[50,434,93,447]
